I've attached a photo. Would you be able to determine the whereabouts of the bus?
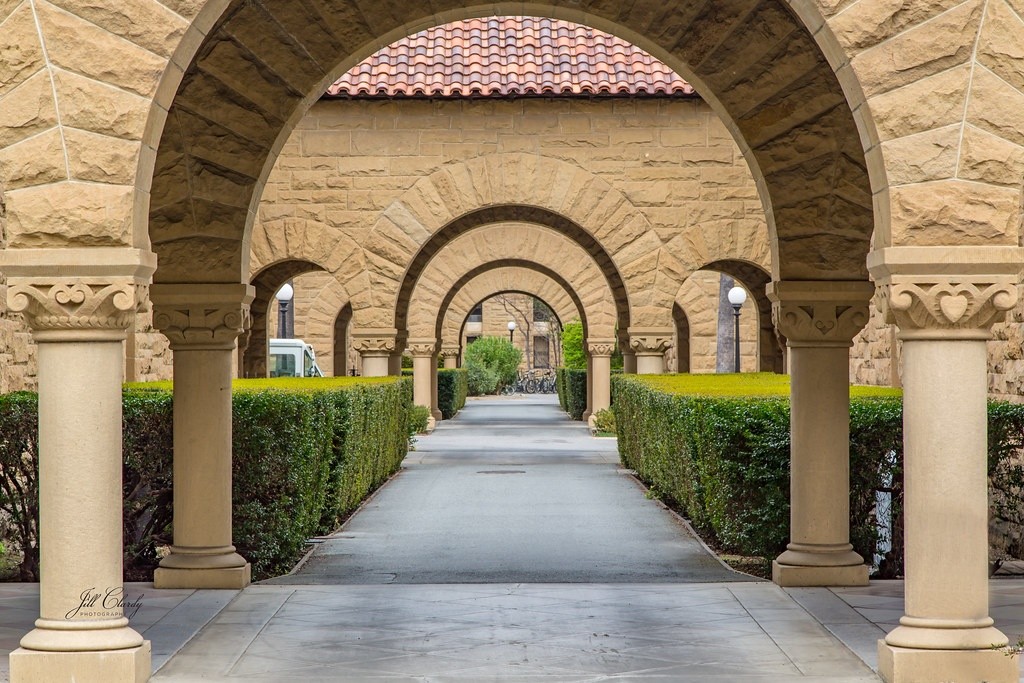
[269,338,325,378]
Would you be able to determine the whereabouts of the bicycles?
[498,366,559,396]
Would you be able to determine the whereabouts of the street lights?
[728,286,746,374]
[276,283,294,378]
[507,321,516,361]
